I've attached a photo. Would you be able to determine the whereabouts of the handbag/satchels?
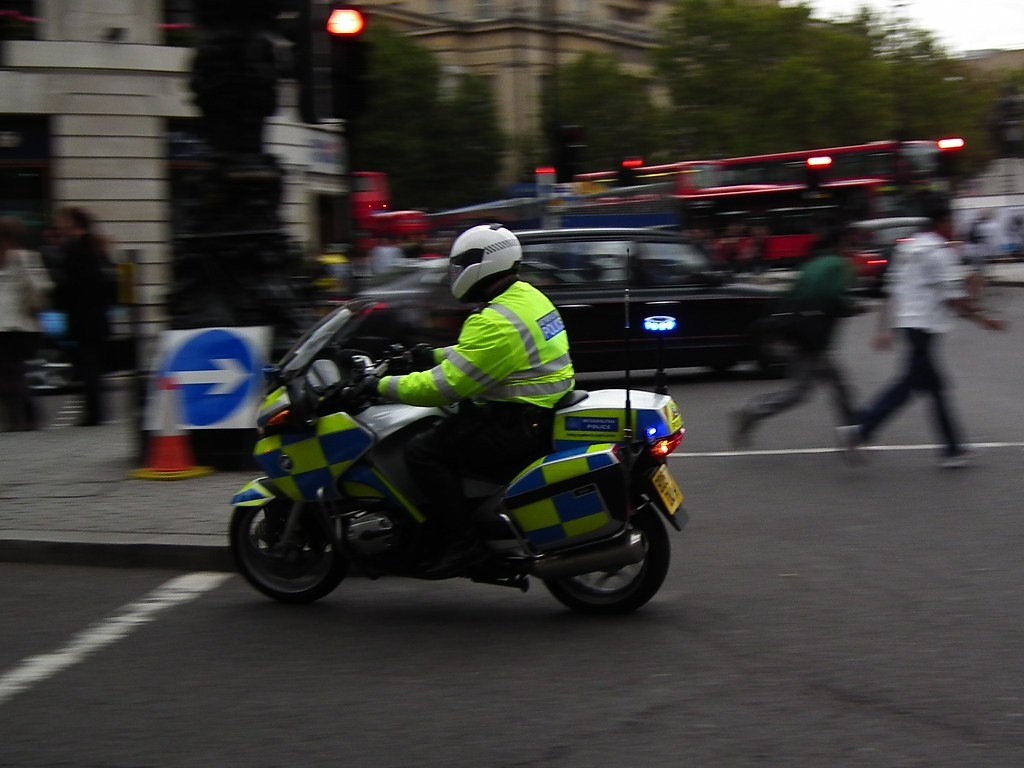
[784,297,832,343]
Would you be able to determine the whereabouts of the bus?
[575,130,964,301]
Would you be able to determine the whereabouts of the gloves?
[409,343,434,371]
[341,355,376,396]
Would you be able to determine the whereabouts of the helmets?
[449,223,522,304]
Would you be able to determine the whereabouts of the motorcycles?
[226,246,695,617]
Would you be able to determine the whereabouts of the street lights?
[326,0,365,300]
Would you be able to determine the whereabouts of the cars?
[308,222,818,386]
[803,213,939,311]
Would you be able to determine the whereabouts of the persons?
[369,235,404,287]
[729,236,867,449]
[348,222,574,568]
[42,206,118,426]
[850,201,1011,468]
[1,215,54,432]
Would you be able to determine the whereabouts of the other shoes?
[836,424,871,464]
[937,453,975,468]
[414,528,480,572]
